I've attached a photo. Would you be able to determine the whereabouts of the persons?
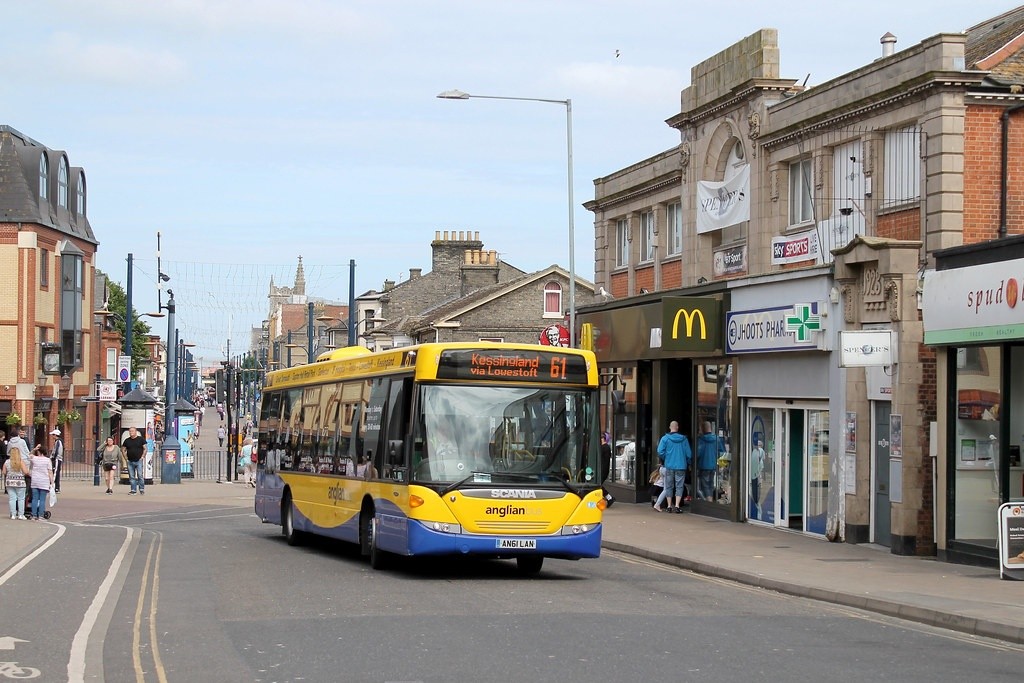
[122,427,148,495]
[256,442,403,479]
[154,394,254,450]
[565,426,616,508]
[146,422,154,443]
[0,427,63,522]
[697,421,774,505]
[651,422,692,513]
[424,413,466,460]
[96,437,123,494]
[619,435,635,483]
[240,438,257,488]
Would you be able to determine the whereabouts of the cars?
[608,440,632,479]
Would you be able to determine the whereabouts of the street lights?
[93,309,167,394]
[436,90,576,350]
[142,338,201,409]
[285,344,337,364]
[316,315,386,346]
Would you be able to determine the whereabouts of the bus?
[254,345,624,576]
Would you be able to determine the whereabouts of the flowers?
[5,413,21,424]
[58,410,68,424]
[67,410,82,424]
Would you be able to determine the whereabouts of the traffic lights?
[230,448,233,452]
[239,434,242,444]
[230,434,234,446]
[239,446,242,452]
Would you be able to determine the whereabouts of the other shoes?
[128,491,137,496]
[18,515,27,520]
[38,517,47,522]
[105,488,113,495]
[666,506,672,513]
[55,488,60,493]
[139,491,144,495]
[654,504,662,512]
[673,507,683,513]
[606,497,616,507]
[32,517,39,522]
[10,516,16,519]
[251,481,256,488]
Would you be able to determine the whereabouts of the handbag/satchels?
[649,469,660,484]
[49,488,57,507]
[105,463,116,470]
[251,446,257,463]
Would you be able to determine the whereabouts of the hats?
[49,430,61,436]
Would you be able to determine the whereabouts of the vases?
[10,424,17,436]
